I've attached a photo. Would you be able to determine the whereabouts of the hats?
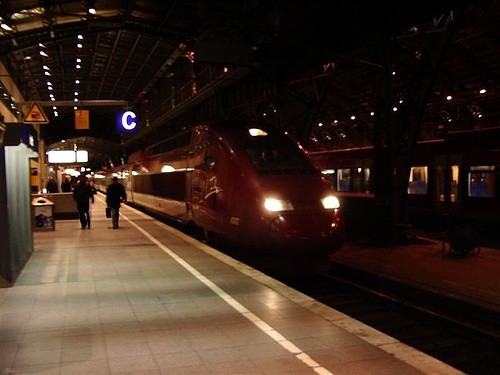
[112,177,118,181]
[77,174,88,181]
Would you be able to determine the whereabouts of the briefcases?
[106,206,111,218]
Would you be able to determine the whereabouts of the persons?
[319,165,500,199]
[32,170,98,195]
[106,176,127,229]
[73,173,94,230]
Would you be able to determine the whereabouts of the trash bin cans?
[32,197,56,232]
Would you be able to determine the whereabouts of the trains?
[86,118,346,261]
